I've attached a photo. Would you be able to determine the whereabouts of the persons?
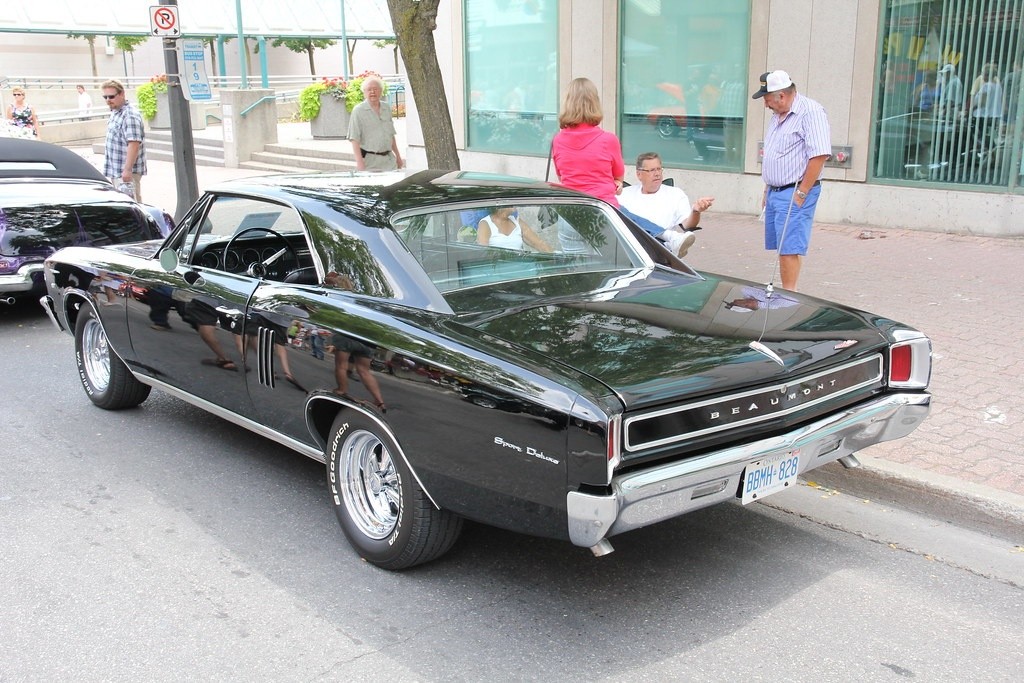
[551,78,624,260]
[84,259,142,308]
[683,67,701,145]
[875,54,1024,168]
[6,87,40,140]
[321,273,387,412]
[753,70,832,292]
[721,285,801,313]
[614,153,714,260]
[76,84,92,121]
[477,205,555,253]
[701,73,722,130]
[502,81,530,140]
[346,76,403,174]
[101,80,147,204]
[147,275,311,394]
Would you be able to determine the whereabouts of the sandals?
[216,359,238,371]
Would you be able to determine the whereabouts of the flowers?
[137,74,168,118]
[300,71,388,121]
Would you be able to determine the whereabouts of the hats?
[940,64,954,71]
[752,69,795,100]
[720,300,754,312]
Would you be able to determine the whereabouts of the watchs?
[796,190,806,199]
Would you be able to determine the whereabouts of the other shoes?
[151,323,171,332]
[347,372,360,382]
[286,372,309,395]
[378,402,387,413]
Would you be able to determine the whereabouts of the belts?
[770,181,819,191]
[365,150,391,155]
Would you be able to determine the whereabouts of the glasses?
[102,91,118,100]
[13,94,23,96]
[635,167,663,173]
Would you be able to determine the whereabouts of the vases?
[148,94,170,130]
[310,95,388,139]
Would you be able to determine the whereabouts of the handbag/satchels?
[538,204,558,228]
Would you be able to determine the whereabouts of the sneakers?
[668,231,694,258]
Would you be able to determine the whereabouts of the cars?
[695,116,938,178]
[876,110,1024,186]
[38,171,934,570]
[0,136,179,305]
[646,83,726,138]
